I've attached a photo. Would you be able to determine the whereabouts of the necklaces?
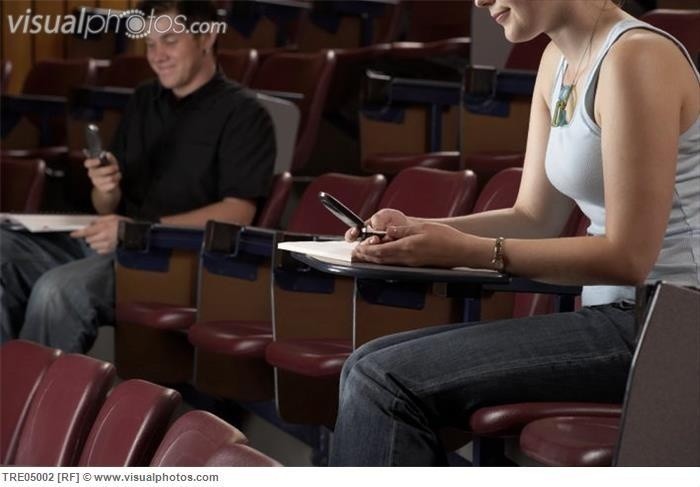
[550,0,609,127]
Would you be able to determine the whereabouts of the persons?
[331,0,700,467]
[0,0,276,355]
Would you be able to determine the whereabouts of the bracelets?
[134,215,161,224]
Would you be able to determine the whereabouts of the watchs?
[491,236,505,274]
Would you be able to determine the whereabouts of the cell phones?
[319,192,387,243]
[85,124,108,168]
